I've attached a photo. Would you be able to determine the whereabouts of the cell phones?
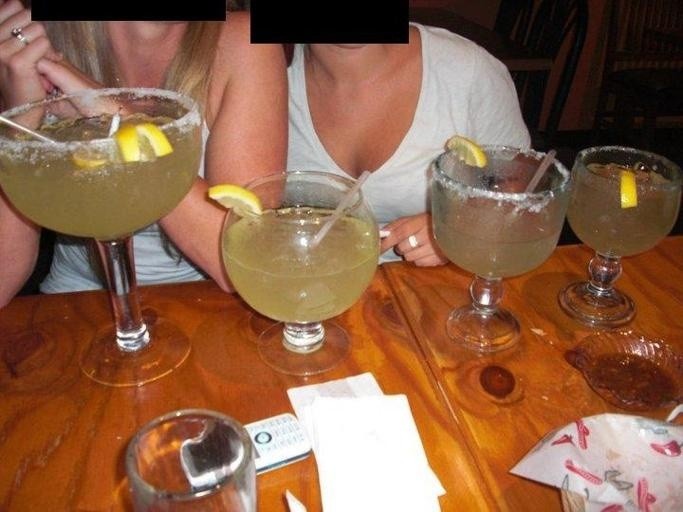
[180,413,311,498]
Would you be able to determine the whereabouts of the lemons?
[209,184,262,221]
[617,170,639,210]
[112,122,139,162]
[444,135,487,168]
[74,139,108,166]
[134,124,173,158]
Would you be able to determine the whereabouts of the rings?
[409,235,418,248]
[13,28,29,46]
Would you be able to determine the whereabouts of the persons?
[0,0,289,309]
[287,23,547,268]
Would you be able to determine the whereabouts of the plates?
[568,329,682,413]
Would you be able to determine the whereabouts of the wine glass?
[569,145,682,326]
[427,144,572,355]
[0,86,203,386]
[220,170,380,376]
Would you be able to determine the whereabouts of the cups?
[122,408,259,512]
